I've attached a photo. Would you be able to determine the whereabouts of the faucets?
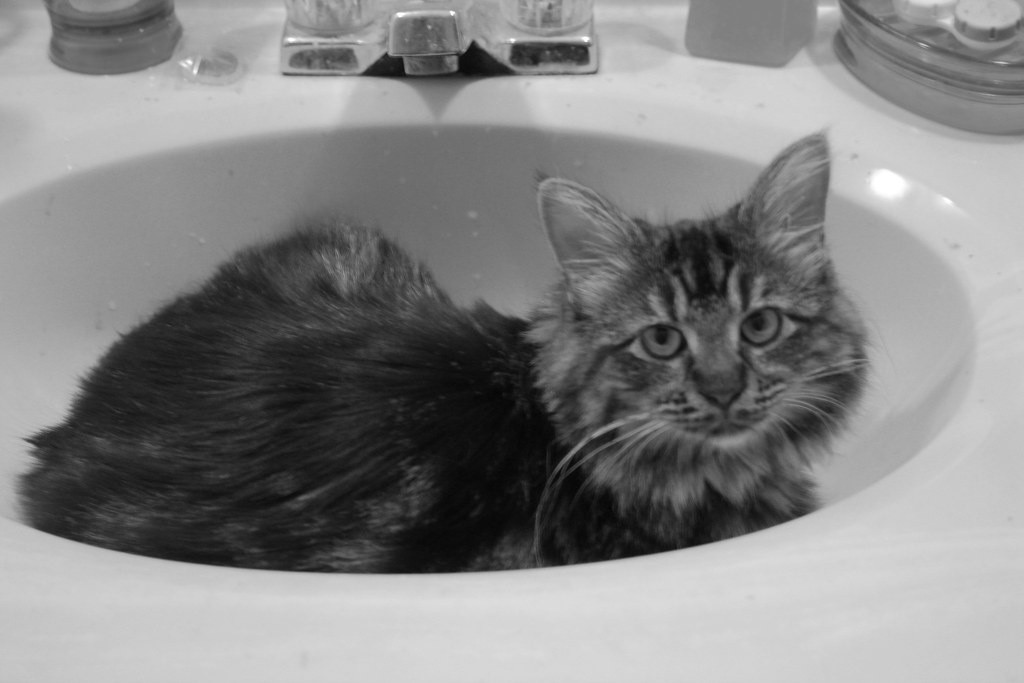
[386,0,476,76]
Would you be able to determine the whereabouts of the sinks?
[0,116,996,580]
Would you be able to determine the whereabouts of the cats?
[17,129,871,581]
